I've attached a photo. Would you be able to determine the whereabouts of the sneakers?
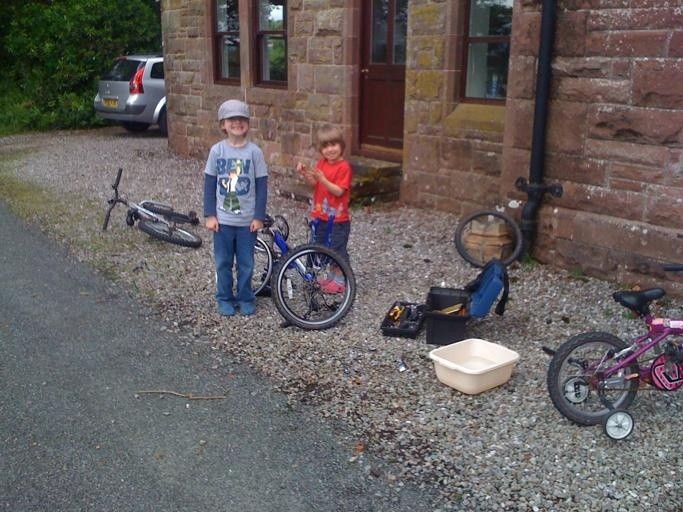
[239,301,257,316]
[217,300,235,316]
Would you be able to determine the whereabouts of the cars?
[94,53,169,137]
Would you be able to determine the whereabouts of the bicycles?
[545,264,683,441]
[214,213,357,330]
[101,168,203,249]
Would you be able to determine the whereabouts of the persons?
[292,124,352,294]
[199,97,268,317]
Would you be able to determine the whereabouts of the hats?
[217,99,250,121]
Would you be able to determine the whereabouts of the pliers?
[389,303,405,320]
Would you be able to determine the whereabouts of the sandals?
[315,275,346,294]
